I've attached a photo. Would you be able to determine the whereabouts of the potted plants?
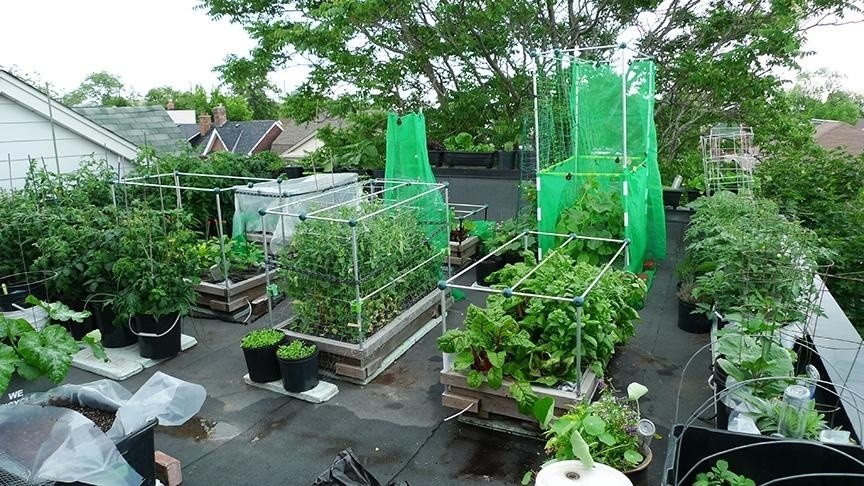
[276,339,319,393]
[0,187,212,360]
[505,215,538,265]
[240,328,286,383]
[470,221,505,286]
[534,377,661,486]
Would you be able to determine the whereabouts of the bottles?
[631,419,656,459]
[777,385,809,442]
